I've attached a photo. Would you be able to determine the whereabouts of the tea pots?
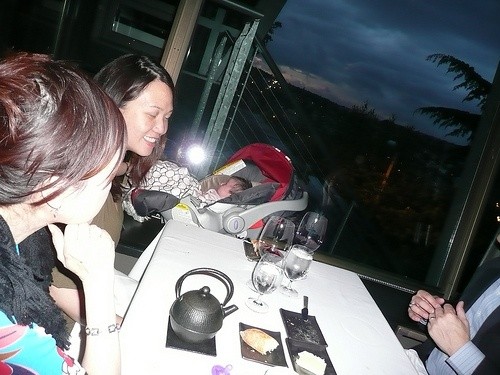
[170,268,239,344]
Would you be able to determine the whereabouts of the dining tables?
[115,219,418,375]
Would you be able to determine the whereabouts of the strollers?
[159,143,310,244]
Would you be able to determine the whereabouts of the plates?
[286,337,337,375]
[279,308,328,348]
[165,318,217,357]
[238,321,289,368]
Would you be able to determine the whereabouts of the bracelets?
[86,323,120,335]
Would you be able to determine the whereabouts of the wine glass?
[280,244,314,298]
[245,253,287,313]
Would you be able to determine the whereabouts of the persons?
[49,54,176,328]
[204,176,253,205]
[409,234,500,375]
[0,52,127,375]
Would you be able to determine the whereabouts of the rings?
[429,317,436,321]
[409,303,416,309]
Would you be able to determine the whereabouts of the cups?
[258,216,295,264]
[296,212,328,255]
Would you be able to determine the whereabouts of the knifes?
[302,296,309,322]
